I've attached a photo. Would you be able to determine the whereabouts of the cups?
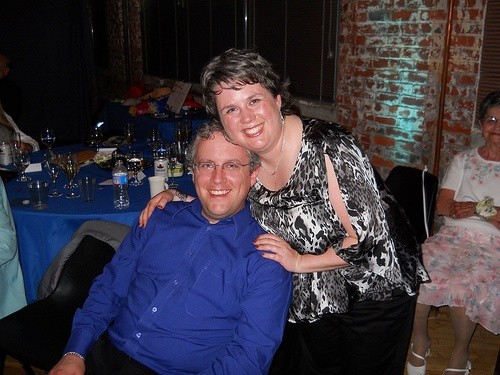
[26,179,51,210]
[154,159,169,177]
[81,177,97,202]
[83,127,96,147]
[169,163,183,177]
[120,122,136,149]
[148,177,169,199]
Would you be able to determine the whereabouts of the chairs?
[384,166,439,254]
[0,221,131,375]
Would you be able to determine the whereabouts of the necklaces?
[261,123,286,174]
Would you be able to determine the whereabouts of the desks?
[4,102,218,306]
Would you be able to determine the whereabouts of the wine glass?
[148,118,192,157]
[175,141,186,177]
[41,128,56,160]
[57,151,80,198]
[45,149,63,197]
[167,152,179,188]
[13,149,32,182]
[92,127,103,153]
[126,150,144,186]
[9,133,21,151]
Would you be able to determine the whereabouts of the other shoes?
[407,345,431,374]
[443,360,471,375]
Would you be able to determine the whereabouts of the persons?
[0,51,39,156]
[49,121,293,375]
[0,175,28,375]
[140,49,431,375]
[406,89,500,375]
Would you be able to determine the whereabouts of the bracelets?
[64,352,84,360]
[177,192,187,202]
[476,196,496,219]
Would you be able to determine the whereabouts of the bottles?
[112,160,129,210]
[0,141,12,168]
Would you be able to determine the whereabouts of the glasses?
[194,161,251,175]
[483,117,500,126]
